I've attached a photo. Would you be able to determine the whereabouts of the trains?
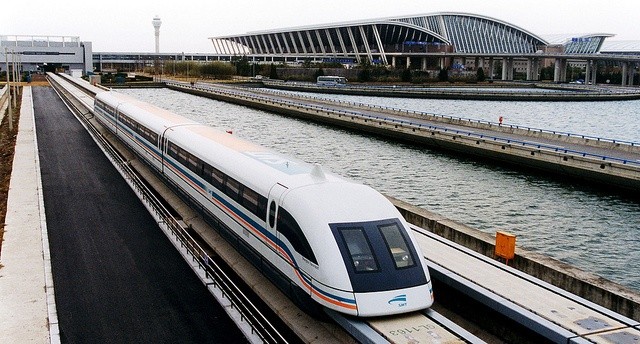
[93,91,435,318]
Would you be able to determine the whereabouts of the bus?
[317,76,348,88]
